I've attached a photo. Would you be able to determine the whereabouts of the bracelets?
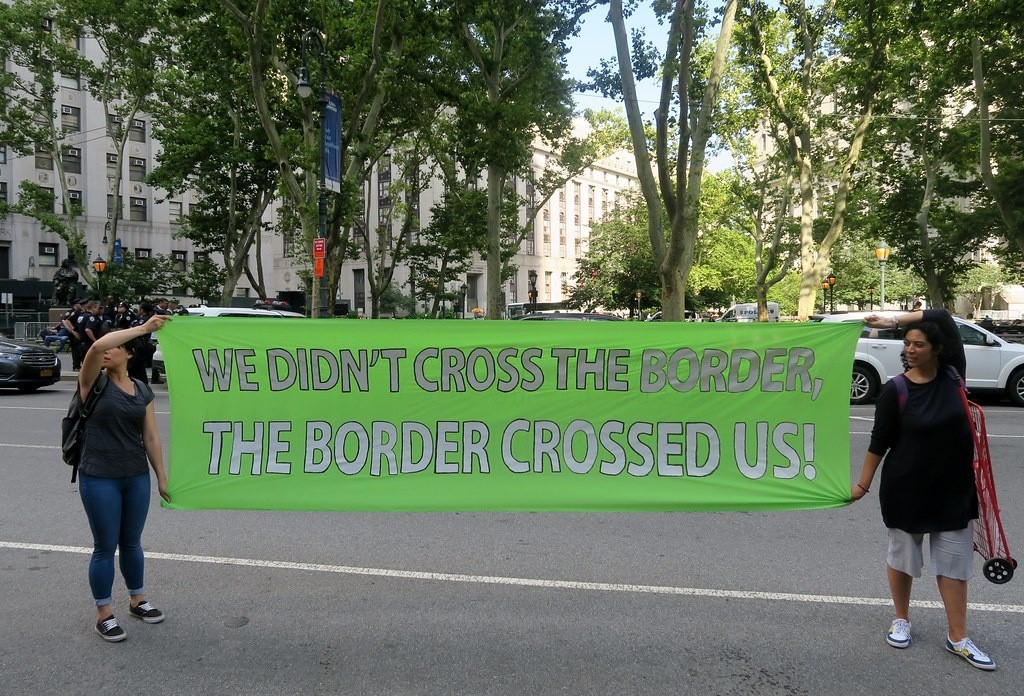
[856,482,869,493]
[891,316,899,329]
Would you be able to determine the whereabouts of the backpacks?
[61,369,149,466]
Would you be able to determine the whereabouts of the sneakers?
[129,601,165,624]
[886,618,913,648]
[945,633,996,670]
[95,614,127,642]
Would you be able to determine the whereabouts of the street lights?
[868,286,875,311]
[298,66,327,318]
[827,273,837,312]
[635,288,641,321]
[868,286,874,309]
[460,282,468,319]
[529,269,539,313]
[822,279,829,312]
[875,237,891,309]
[94,254,106,291]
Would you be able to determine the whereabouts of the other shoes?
[151,380,164,384]
[55,350,59,355]
[73,366,81,372]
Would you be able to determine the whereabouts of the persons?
[77,315,171,642]
[52,259,78,306]
[845,302,996,670]
[43,291,189,384]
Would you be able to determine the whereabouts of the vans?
[715,303,781,322]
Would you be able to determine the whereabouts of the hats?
[77,299,89,305]
[99,304,104,309]
[118,301,128,308]
[73,299,80,304]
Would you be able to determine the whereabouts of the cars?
[151,304,307,385]
[804,310,1024,407]
[507,313,625,321]
[644,310,705,322]
[0,333,62,391]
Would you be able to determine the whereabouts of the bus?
[508,301,585,320]
